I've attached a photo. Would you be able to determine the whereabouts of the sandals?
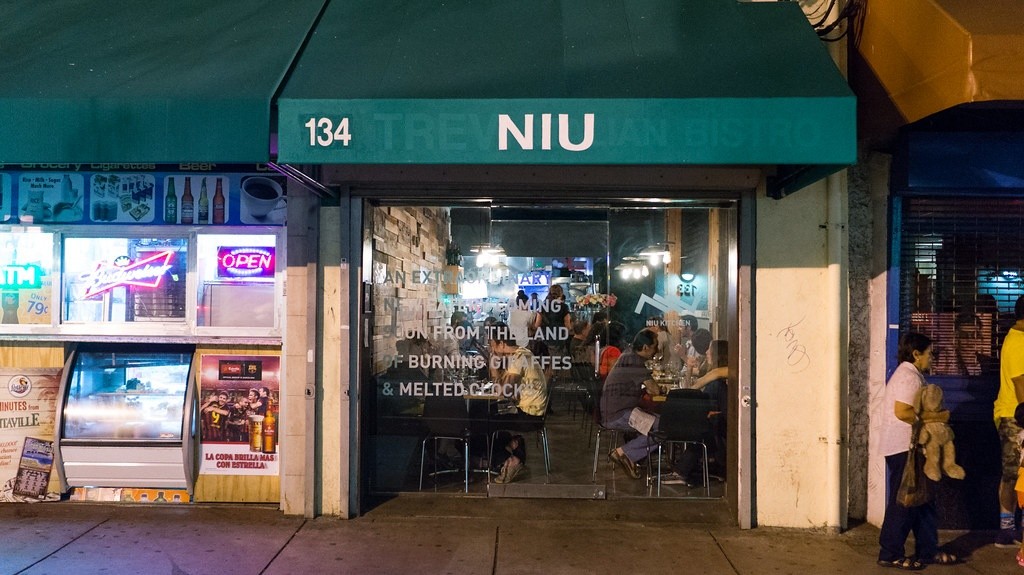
[877,557,923,571]
[921,552,957,565]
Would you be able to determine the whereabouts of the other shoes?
[1016,553,1024,566]
[995,529,1023,549]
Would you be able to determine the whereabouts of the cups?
[680,377,691,388]
[248,414,265,451]
[69,189,80,202]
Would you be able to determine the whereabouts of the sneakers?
[511,435,525,464]
[660,473,688,486]
[494,461,523,483]
[609,447,642,480]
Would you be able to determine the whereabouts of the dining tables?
[463,394,503,414]
[645,358,684,401]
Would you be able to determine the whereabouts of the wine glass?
[447,363,469,385]
[669,360,679,388]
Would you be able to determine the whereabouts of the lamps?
[613,242,671,281]
[470,244,510,285]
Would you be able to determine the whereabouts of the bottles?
[164,176,226,225]
[261,398,277,454]
[61,173,72,200]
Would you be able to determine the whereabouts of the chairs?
[419,385,472,494]
[567,361,653,484]
[489,373,559,480]
[646,389,710,498]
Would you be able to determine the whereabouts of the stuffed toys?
[913,382,968,484]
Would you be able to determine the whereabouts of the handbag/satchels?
[896,443,938,507]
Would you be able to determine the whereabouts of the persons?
[876,329,959,571]
[380,279,606,473]
[647,315,681,372]
[201,384,273,446]
[991,292,1024,553]
[683,328,715,387]
[1012,400,1024,567]
[674,315,700,377]
[599,327,671,481]
[598,319,628,381]
[664,338,729,485]
